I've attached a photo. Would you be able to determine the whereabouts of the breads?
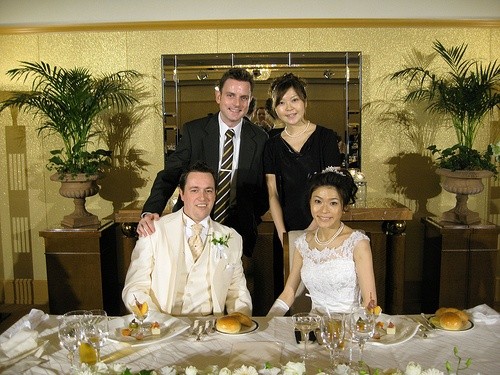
[216,312,253,333]
[436,307,468,331]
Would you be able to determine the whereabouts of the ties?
[188,224,205,262]
[213,129,235,225]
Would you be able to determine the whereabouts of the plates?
[108,310,191,347]
[344,312,421,347]
[426,316,474,332]
[212,319,259,336]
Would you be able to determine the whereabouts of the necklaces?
[314,221,345,245]
[284,120,310,138]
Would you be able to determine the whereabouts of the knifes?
[421,313,437,330]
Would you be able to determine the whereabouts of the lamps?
[246,69,271,80]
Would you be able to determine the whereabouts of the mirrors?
[161,50,361,183]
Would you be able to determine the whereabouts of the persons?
[122,158,253,316]
[266,166,377,318]
[136,67,270,316]
[262,73,343,315]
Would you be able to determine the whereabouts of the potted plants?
[0,60,140,228]
[391,39,500,226]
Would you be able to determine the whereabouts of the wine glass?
[291,312,321,366]
[58,310,108,375]
[133,297,148,334]
[320,313,345,375]
[350,311,376,375]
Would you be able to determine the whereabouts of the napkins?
[473,311,499,325]
[0,329,38,358]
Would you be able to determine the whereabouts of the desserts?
[356,317,396,335]
[122,319,160,337]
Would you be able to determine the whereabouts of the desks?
[38,220,119,317]
[117,199,413,315]
[0,304,500,375]
[422,216,500,315]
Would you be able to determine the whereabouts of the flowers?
[74,346,482,375]
